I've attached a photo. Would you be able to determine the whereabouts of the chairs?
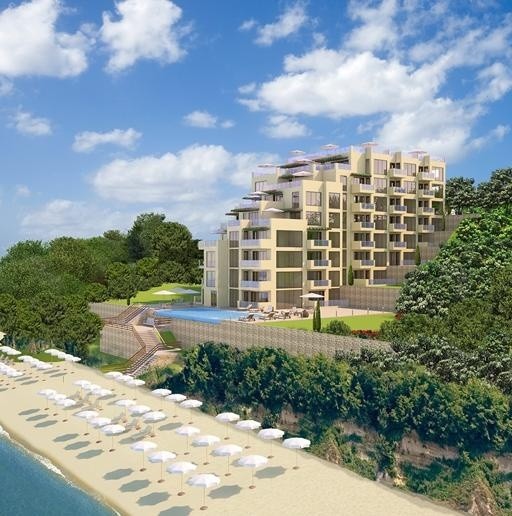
[237,303,309,324]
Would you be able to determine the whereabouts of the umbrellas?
[153,290,175,295]
[170,288,188,293]
[408,150,426,154]
[298,292,326,298]
[0,346,81,378]
[38,370,310,511]
[289,150,305,154]
[248,191,268,196]
[361,142,378,147]
[256,164,272,168]
[262,207,283,214]
[186,288,199,294]
[292,170,313,177]
[293,159,312,163]
[321,144,338,150]
